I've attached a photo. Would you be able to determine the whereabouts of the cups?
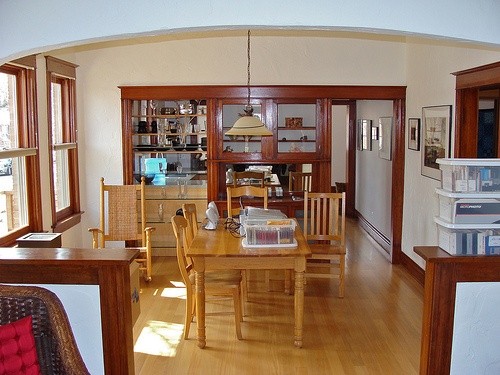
[177,166,182,174]
[201,137,207,150]
[193,124,201,133]
[186,125,192,133]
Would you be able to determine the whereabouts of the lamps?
[223,28,274,136]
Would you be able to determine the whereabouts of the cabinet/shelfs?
[222,104,261,153]
[215,201,331,265]
[277,103,317,152]
[122,98,209,256]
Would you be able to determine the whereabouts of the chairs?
[170,203,248,340]
[288,171,312,220]
[87,177,156,282]
[226,187,347,299]
[233,171,265,187]
[0,284,91,375]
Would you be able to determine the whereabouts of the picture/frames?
[408,118,420,151]
[421,105,452,181]
[356,116,393,162]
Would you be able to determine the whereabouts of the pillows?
[0,316,42,375]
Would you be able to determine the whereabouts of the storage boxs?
[434,157,500,255]
[244,219,298,243]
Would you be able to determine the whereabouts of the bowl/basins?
[134,173,156,184]
[137,143,200,151]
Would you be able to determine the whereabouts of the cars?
[0,159,12,176]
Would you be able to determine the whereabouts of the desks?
[226,174,281,186]
[186,217,312,349]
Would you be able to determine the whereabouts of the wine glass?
[151,99,158,116]
